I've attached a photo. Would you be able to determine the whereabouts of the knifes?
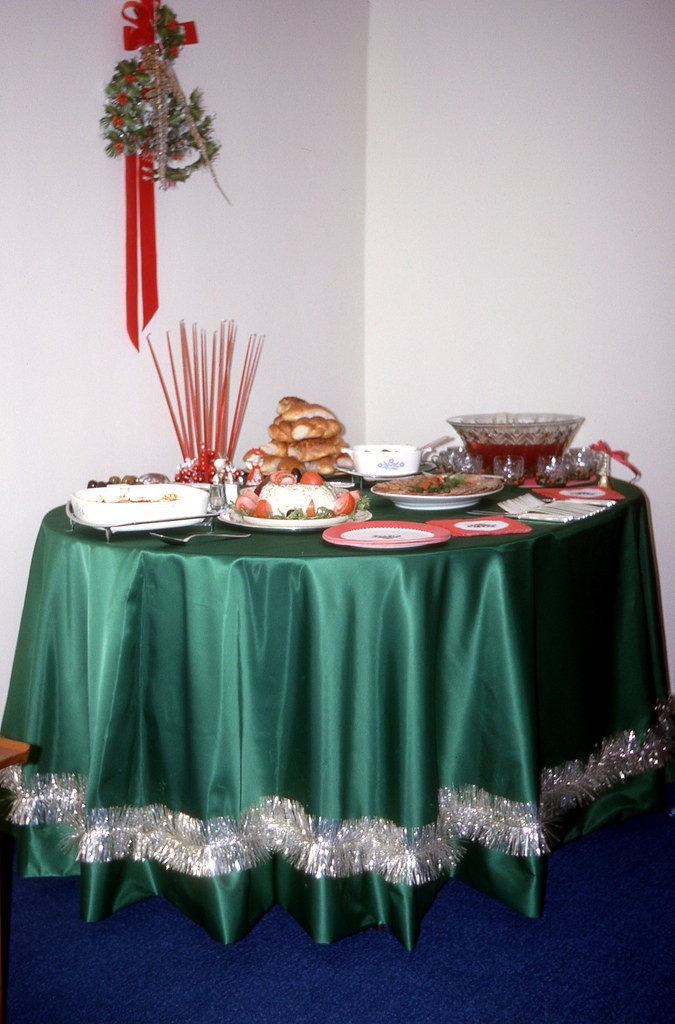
[466,510,573,522]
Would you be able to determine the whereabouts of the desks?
[0,472,675,953]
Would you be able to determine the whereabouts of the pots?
[70,482,209,533]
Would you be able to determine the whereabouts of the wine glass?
[210,484,227,535]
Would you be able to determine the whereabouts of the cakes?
[234,470,359,519]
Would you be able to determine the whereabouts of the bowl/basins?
[341,443,422,475]
[370,475,504,510]
[445,411,585,478]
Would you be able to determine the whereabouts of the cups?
[569,447,591,479]
[460,455,482,473]
[432,450,448,466]
[493,455,525,485]
[537,455,569,487]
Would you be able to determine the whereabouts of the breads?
[258,395,352,475]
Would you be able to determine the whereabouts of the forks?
[497,494,606,520]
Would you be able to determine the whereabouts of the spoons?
[148,531,252,543]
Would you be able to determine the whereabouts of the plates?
[353,472,433,481]
[322,520,452,551]
[217,507,373,532]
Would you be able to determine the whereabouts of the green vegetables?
[407,468,460,494]
